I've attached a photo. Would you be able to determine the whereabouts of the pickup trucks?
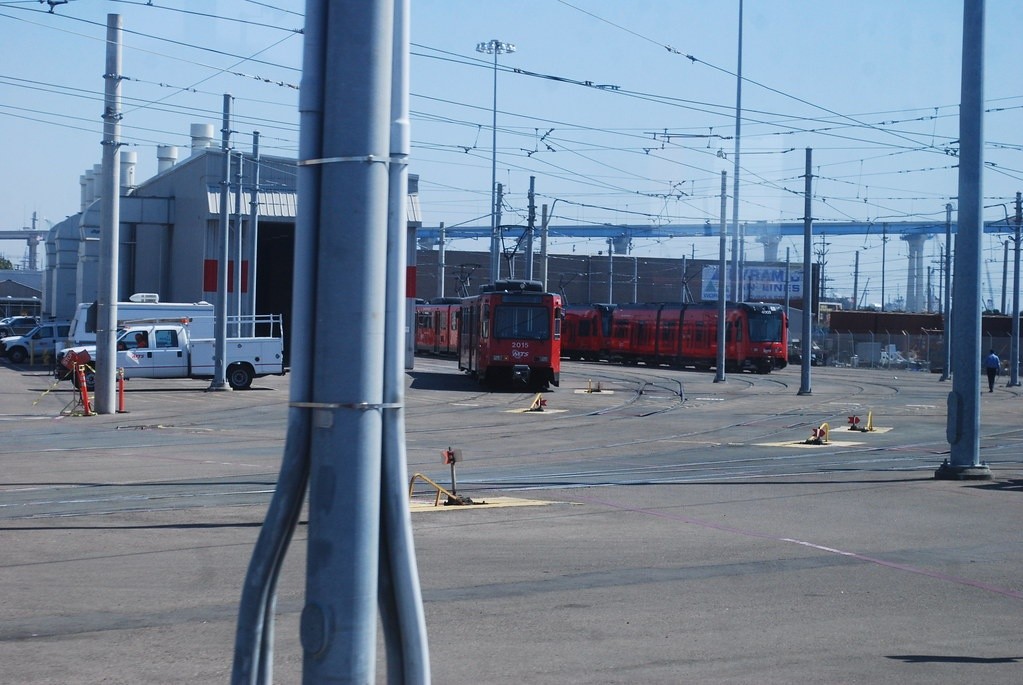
[57,315,283,391]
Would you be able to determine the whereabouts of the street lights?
[477,39,516,280]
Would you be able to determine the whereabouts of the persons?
[135,334,147,349]
[986,350,1001,393]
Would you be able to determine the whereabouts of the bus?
[406,297,789,374]
[458,280,562,391]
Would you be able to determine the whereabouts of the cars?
[0,316,40,339]
[0,322,70,363]
